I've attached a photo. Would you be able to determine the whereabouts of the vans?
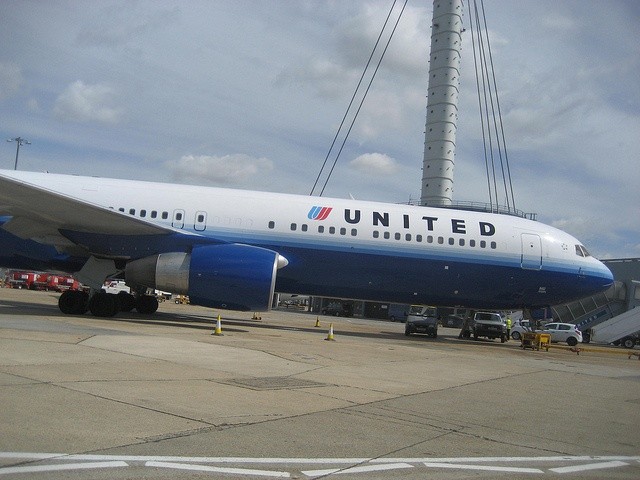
[320,302,353,318]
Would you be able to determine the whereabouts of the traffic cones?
[324,322,336,342]
[313,316,321,326]
[211,313,225,337]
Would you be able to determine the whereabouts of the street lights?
[6,136,32,170]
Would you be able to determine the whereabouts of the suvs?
[534,322,583,347]
[510,320,532,340]
[100,279,130,294]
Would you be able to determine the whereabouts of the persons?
[505,315,511,340]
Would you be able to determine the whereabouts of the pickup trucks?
[471,311,508,344]
[404,304,439,338]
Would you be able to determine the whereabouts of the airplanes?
[0,167,616,321]
